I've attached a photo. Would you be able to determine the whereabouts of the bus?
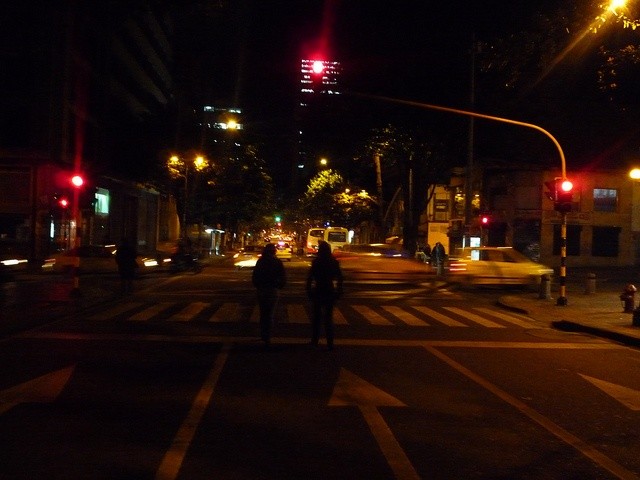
[303,227,325,258]
[321,227,349,261]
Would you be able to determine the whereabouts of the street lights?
[170,154,204,250]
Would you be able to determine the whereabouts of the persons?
[306,241,344,352]
[114,238,139,293]
[423,245,430,257]
[248,244,288,344]
[432,242,446,273]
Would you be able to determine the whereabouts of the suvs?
[271,241,293,261]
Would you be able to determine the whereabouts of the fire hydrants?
[619,284,637,313]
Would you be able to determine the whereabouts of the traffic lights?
[543,180,557,211]
[557,179,572,213]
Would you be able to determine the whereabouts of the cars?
[41,244,172,272]
[234,244,266,271]
[446,247,553,298]
